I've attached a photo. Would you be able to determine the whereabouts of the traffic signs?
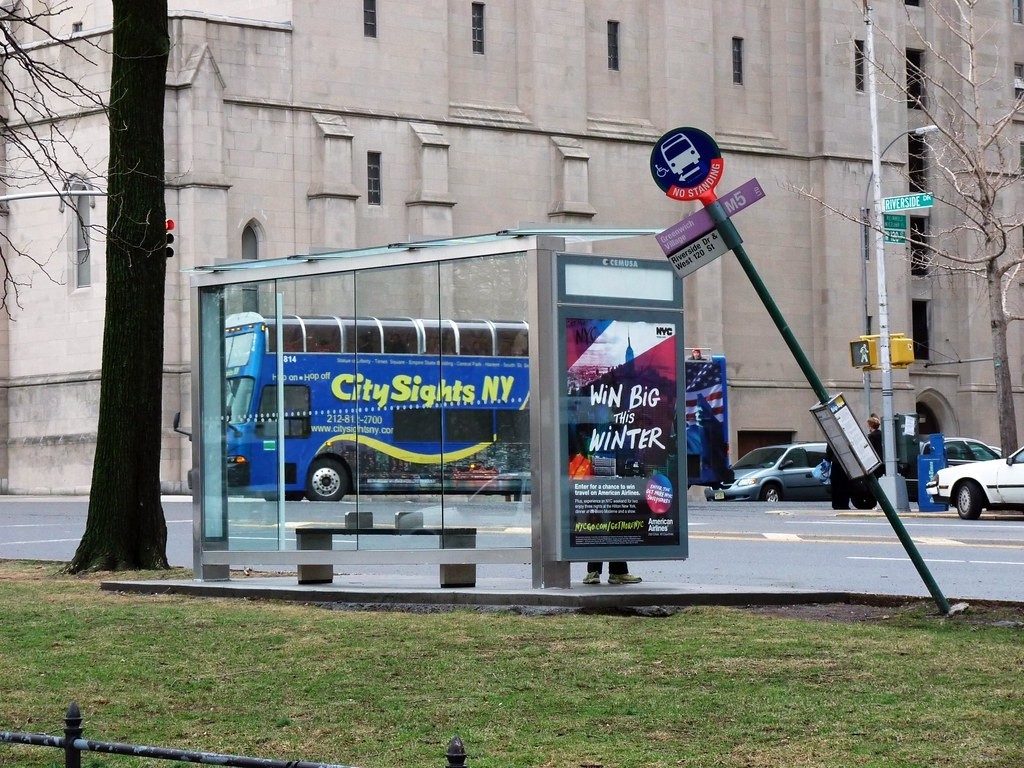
[882,193,934,243]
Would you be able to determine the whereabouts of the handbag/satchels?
[812,459,832,486]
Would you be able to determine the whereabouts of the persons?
[583,561,642,584]
[689,350,704,360]
[821,441,851,510]
[866,418,884,477]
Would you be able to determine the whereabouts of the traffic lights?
[166,218,175,258]
[849,340,877,369]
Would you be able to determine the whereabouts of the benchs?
[295,512,476,588]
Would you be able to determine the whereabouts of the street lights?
[864,125,940,430]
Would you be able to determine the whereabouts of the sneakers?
[583,571,601,584]
[608,573,642,584]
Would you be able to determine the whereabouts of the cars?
[703,440,832,501]
[988,446,1001,457]
[925,445,1024,519]
[919,435,1001,469]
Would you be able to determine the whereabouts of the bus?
[171,311,531,502]
